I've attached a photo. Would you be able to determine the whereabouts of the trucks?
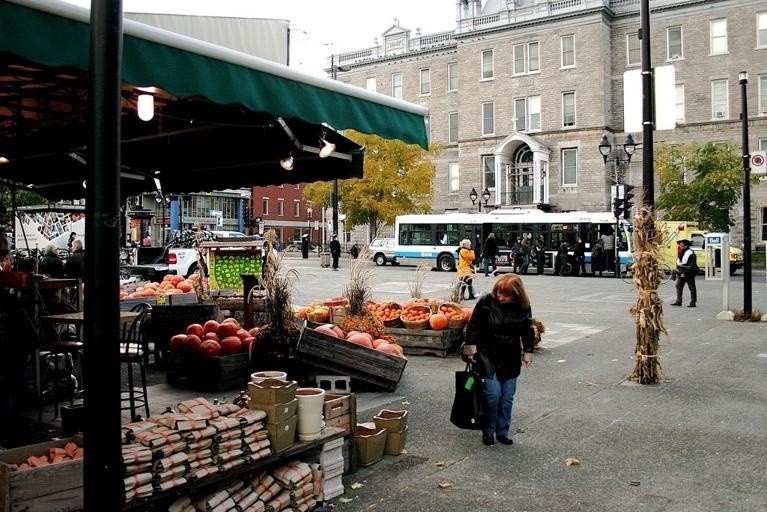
[629,216,745,277]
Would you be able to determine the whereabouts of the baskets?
[374,302,403,327]
[437,302,466,329]
[323,298,346,307]
[400,305,432,331]
[305,309,330,325]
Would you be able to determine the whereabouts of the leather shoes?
[483,433,494,445]
[496,435,513,445]
[687,305,697,307]
[670,303,682,307]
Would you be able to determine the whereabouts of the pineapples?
[282,319,300,331]
[255,324,272,337]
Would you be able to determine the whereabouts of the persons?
[330,234,341,271]
[480,233,501,276]
[440,232,447,245]
[39,244,65,281]
[510,230,614,278]
[669,239,697,307]
[349,245,358,259]
[456,238,479,300]
[67,231,77,253]
[65,238,86,302]
[466,272,536,444]
[0,227,9,262]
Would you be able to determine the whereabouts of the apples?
[440,305,465,320]
[363,303,430,320]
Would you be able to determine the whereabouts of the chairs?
[36,303,83,420]
[120,302,153,423]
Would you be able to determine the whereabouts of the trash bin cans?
[320,252,330,268]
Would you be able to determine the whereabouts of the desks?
[40,309,143,418]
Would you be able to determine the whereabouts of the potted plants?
[249,249,305,382]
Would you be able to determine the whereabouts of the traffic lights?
[623,182,635,219]
[241,197,247,219]
[243,207,249,228]
[613,197,623,220]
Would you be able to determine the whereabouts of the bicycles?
[620,248,673,287]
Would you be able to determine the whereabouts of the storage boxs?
[0,434,84,512]
[324,393,357,435]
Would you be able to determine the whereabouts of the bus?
[392,209,637,280]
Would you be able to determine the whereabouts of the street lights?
[469,186,491,214]
[154,191,172,247]
[599,133,637,281]
[735,70,755,320]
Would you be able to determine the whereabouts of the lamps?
[317,132,336,158]
[280,154,294,170]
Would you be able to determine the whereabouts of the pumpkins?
[314,323,402,356]
[429,314,448,330]
[120,274,197,297]
[464,307,473,323]
[169,318,261,355]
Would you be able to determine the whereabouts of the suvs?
[160,226,398,280]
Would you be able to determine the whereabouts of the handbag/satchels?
[449,359,491,428]
